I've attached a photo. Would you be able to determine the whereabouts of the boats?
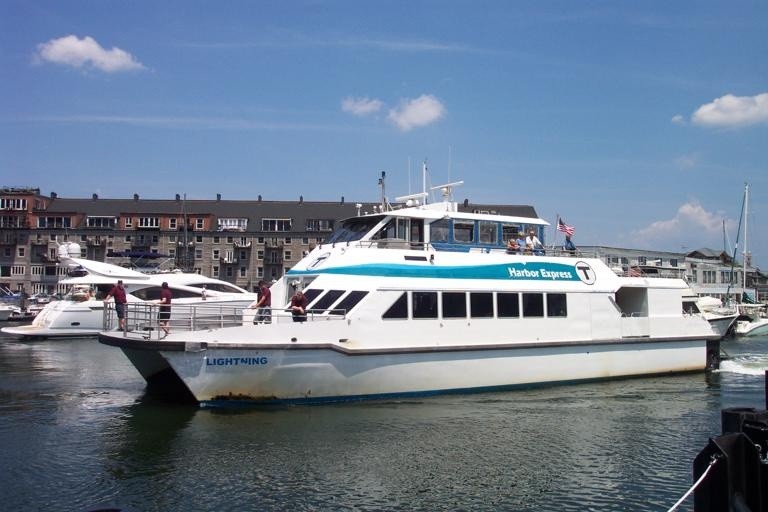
[0,286,60,320]
[0,251,258,343]
[95,144,721,406]
[614,180,767,339]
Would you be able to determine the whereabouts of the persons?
[104,279,129,331]
[561,233,581,257]
[516,231,532,255]
[150,281,172,340]
[88,288,97,301]
[525,230,548,256]
[505,238,520,255]
[290,289,310,322]
[202,285,207,300]
[250,280,272,325]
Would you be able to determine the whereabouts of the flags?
[556,216,576,238]
[624,264,640,277]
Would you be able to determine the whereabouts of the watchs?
[542,247,544,249]
